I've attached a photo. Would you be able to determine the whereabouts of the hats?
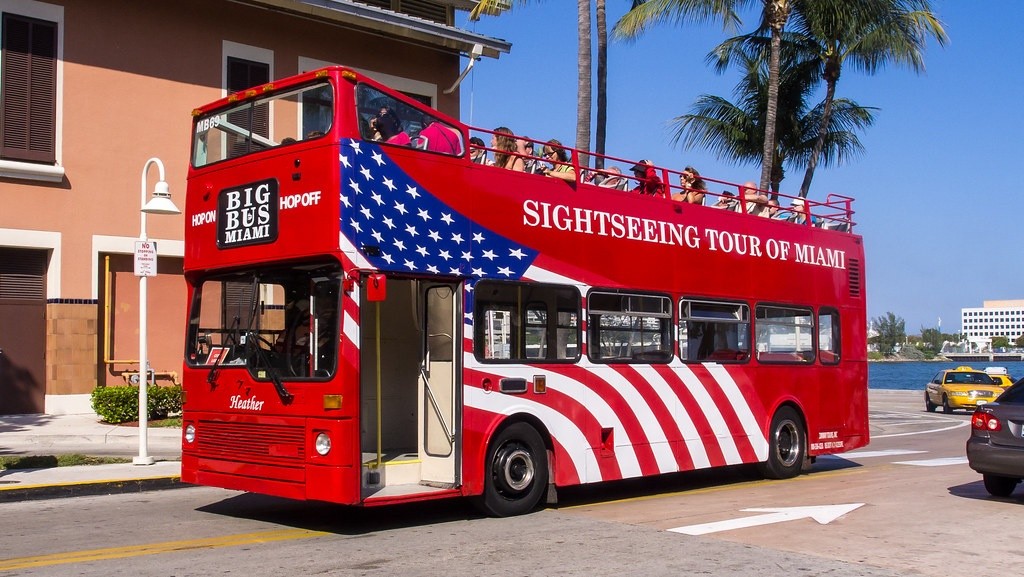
[790,197,805,206]
[629,159,656,173]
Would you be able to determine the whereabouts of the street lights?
[134,158,182,464]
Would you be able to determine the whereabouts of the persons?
[271,301,330,355]
[360,107,820,226]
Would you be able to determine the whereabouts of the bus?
[180,65,870,517]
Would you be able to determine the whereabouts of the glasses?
[543,151,556,157]
[375,113,389,119]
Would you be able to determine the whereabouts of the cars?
[966,378,1024,497]
[924,365,1006,413]
[981,367,1017,389]
